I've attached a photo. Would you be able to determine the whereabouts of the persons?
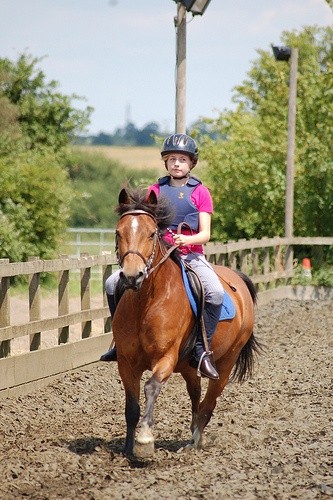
[99,134,224,381]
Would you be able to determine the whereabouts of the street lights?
[170,0,211,133]
[271,47,298,236]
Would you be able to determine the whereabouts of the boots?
[100,292,118,361]
[188,301,224,380]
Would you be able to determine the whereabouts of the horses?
[113,178,267,467]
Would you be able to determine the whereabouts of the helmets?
[160,134,200,163]
